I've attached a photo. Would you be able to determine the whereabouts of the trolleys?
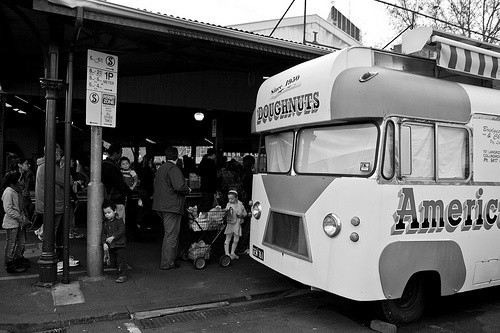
[183,199,233,271]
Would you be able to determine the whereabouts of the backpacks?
[223,160,248,204]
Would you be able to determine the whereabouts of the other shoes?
[161,264,179,270]
[231,255,239,260]
[34,228,44,241]
[115,275,127,282]
[228,255,235,260]
[6,262,26,273]
[69,256,79,266]
[57,261,63,272]
[69,228,84,238]
[15,259,30,267]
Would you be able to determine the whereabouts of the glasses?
[56,149,63,154]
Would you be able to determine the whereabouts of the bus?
[244,27,500,327]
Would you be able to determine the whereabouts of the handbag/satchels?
[17,211,31,230]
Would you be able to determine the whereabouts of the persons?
[100,199,127,283]
[0,142,86,274]
[102,143,256,263]
[153,147,191,270]
[224,189,247,262]
